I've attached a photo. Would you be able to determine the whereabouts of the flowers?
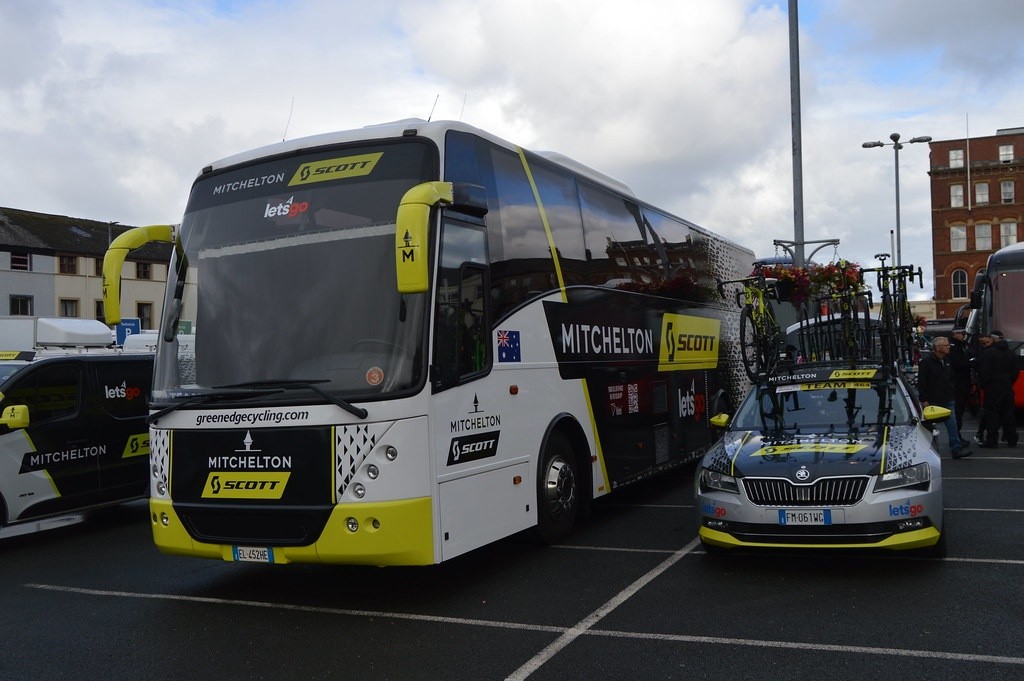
[751,256,862,306]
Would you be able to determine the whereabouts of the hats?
[990,331,1004,339]
[953,326,970,335]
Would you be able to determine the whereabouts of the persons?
[784,345,798,364]
[973,331,1021,447]
[918,337,972,460]
[946,326,973,448]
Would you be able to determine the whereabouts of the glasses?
[937,344,950,348]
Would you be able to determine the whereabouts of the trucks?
[0,315,113,357]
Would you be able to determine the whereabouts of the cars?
[694,359,949,555]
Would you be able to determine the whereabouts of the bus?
[101,119,755,567]
[954,242,1024,423]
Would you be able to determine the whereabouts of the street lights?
[863,133,931,291]
[108,222,120,247]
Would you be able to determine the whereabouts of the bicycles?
[858,253,923,368]
[716,260,876,382]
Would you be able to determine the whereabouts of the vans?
[0,356,155,541]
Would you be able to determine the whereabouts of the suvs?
[757,312,916,364]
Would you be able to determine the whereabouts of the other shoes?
[1009,433,1018,447]
[963,439,970,443]
[979,440,998,448]
[954,448,973,458]
[974,435,984,444]
[962,442,968,446]
